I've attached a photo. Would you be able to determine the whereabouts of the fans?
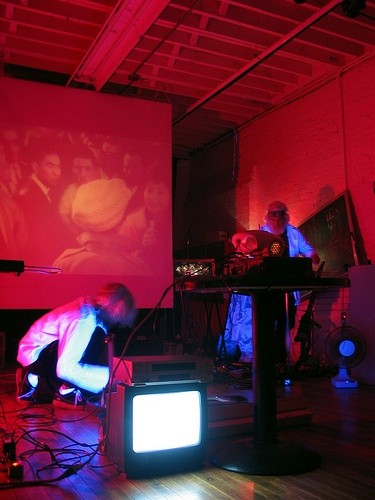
[323,326,368,390]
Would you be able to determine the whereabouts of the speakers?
[105,354,198,473]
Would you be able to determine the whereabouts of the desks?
[191,284,341,476]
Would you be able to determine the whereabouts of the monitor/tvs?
[124,380,208,480]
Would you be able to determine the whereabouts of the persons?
[16,283,135,404]
[260,201,320,330]
[18,145,172,275]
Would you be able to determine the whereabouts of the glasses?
[267,210,286,217]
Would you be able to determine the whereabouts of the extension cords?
[5,451,23,480]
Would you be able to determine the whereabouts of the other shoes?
[15,368,33,405]
[57,387,87,405]
